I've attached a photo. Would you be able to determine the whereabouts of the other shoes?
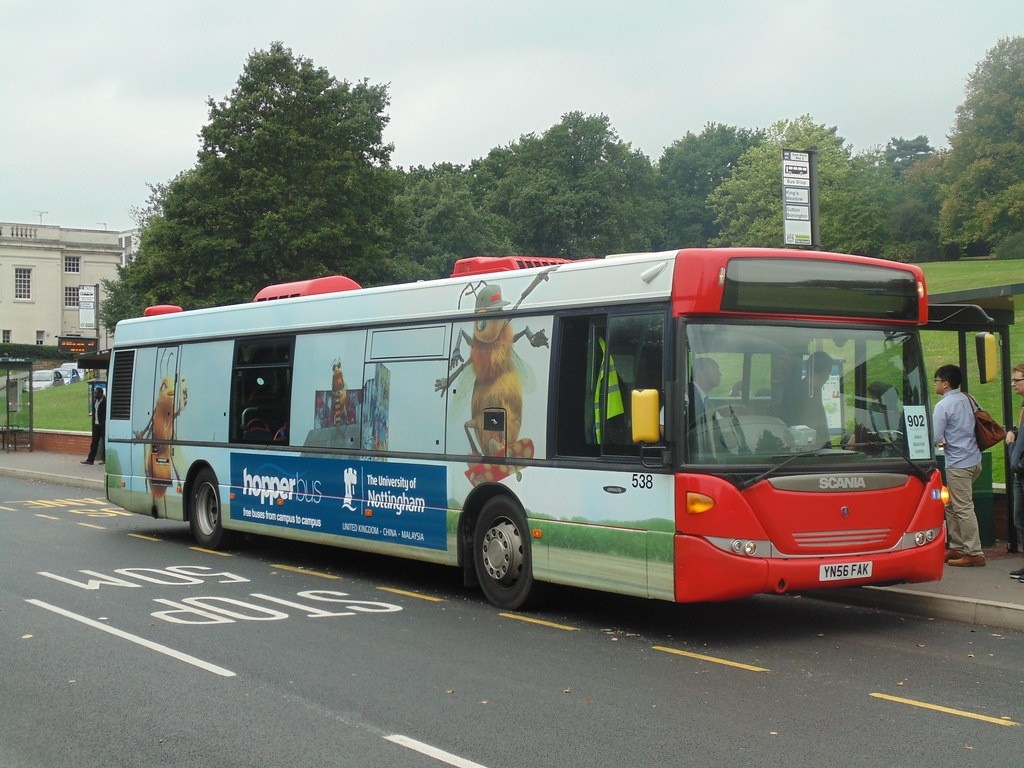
[1018,576,1024,582]
[1010,568,1024,578]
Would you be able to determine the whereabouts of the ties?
[703,396,709,410]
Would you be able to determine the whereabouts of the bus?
[104,244,997,610]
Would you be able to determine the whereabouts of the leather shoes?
[98,462,105,464]
[948,555,986,566]
[81,460,94,465]
[945,550,966,561]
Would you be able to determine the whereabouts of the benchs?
[0,424,24,451]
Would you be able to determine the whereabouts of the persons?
[1003,362,1024,584]
[729,380,753,398]
[840,379,905,459]
[658,357,726,443]
[771,350,835,449]
[928,364,985,568]
[80,387,107,465]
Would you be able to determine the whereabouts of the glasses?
[1011,379,1024,383]
[933,379,945,382]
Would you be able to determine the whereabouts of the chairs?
[244,418,290,444]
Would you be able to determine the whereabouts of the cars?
[61,363,88,382]
[22,369,64,394]
[54,367,80,386]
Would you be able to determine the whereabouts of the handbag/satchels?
[963,392,1007,452]
